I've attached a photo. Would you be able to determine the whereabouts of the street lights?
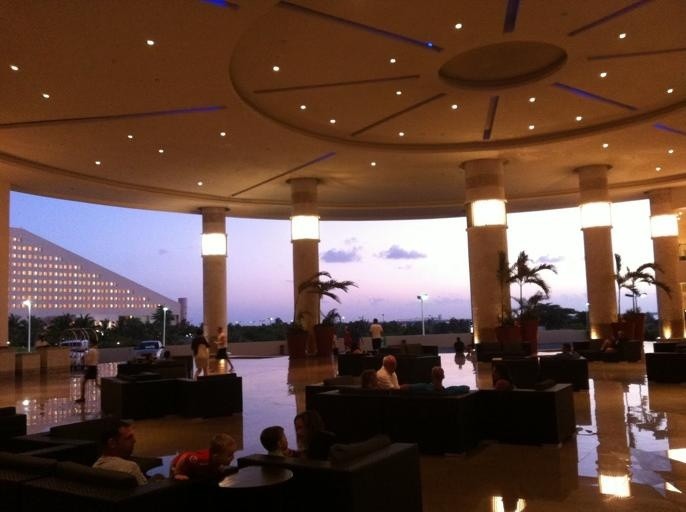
[23,300,32,353]
[417,293,428,336]
[162,307,168,346]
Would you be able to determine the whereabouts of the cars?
[134,341,167,360]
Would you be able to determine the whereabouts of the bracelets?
[172,472,184,476]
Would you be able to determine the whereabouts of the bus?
[59,340,88,351]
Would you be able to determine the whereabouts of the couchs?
[2,406,27,439]
[645,342,684,379]
[236,442,422,510]
[476,339,641,363]
[307,374,483,454]
[20,418,163,477]
[101,355,243,418]
[2,442,239,510]
[493,344,589,390]
[337,344,441,380]
[481,384,574,441]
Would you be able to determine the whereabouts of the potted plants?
[495,245,557,355]
[284,271,361,360]
[609,260,672,354]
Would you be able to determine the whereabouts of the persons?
[357,368,379,390]
[73,337,101,404]
[259,425,293,457]
[34,334,50,349]
[597,333,616,363]
[374,355,411,390]
[209,325,233,373]
[611,329,628,363]
[426,366,447,390]
[168,432,238,483]
[349,326,363,351]
[343,319,352,352]
[453,336,465,354]
[282,409,342,460]
[494,379,518,390]
[88,417,150,487]
[189,326,210,380]
[367,317,384,348]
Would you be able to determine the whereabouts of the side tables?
[216,464,293,510]
